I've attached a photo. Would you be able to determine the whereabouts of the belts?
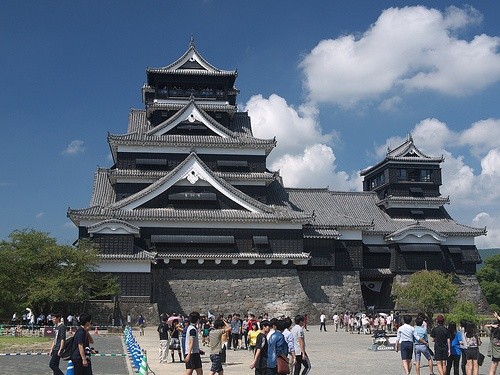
[415,344,425,345]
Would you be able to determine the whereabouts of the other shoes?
[430,373,437,375]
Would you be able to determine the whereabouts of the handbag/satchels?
[276,358,290,374]
[477,353,485,365]
[384,319,387,324]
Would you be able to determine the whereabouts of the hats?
[437,315,444,322]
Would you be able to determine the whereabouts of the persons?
[320,311,327,332]
[250,315,311,375]
[333,311,400,335]
[157,313,243,364]
[135,314,145,336]
[70,313,96,375]
[182,311,205,375]
[49,311,66,375]
[106,313,114,326]
[123,311,133,326]
[12,311,80,331]
[208,319,232,375]
[395,311,500,375]
[240,314,286,359]
[304,314,309,331]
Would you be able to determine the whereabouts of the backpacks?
[60,329,85,360]
